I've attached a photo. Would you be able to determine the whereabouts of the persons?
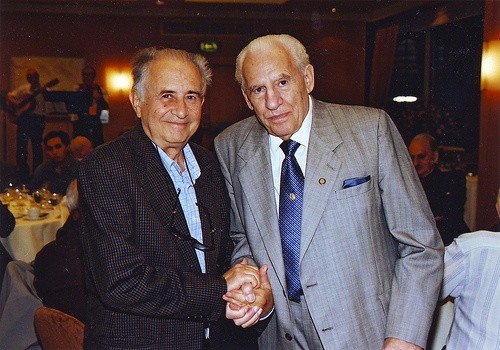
[77,48,275,350]
[1,67,500,350]
[223,35,445,350]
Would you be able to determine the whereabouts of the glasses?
[167,187,216,252]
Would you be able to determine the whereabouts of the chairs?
[0,260,86,350]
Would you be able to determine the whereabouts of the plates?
[22,216,43,220]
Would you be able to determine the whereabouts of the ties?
[278,140,305,300]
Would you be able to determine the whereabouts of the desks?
[0,193,70,264]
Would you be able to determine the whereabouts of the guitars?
[8,78,59,121]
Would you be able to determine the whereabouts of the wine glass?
[2,183,60,217]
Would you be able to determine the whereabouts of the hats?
[72,136,92,160]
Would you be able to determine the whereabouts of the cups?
[28,207,39,217]
